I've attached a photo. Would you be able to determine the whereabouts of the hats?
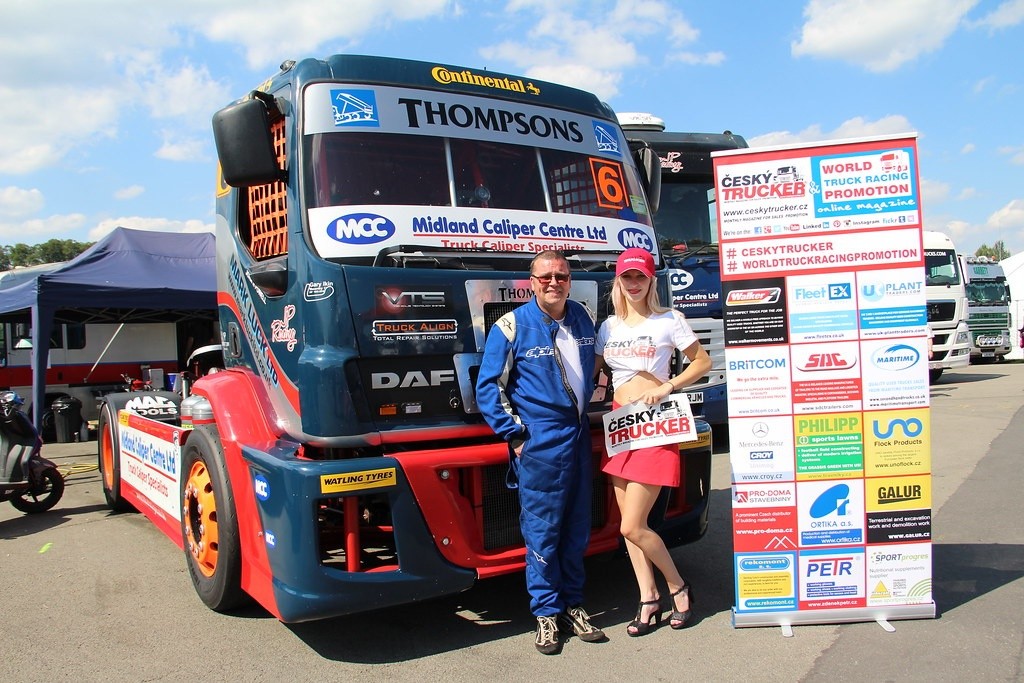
[616,248,656,279]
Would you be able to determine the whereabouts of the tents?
[0,226,217,443]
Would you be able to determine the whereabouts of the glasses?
[531,274,571,283]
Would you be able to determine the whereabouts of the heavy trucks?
[960,254,1014,356]
[614,111,753,423]
[95,51,714,626]
[923,230,972,382]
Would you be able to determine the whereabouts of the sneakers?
[560,606,605,642]
[535,613,560,655]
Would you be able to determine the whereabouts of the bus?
[0,318,225,442]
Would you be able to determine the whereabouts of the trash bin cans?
[51,396,83,443]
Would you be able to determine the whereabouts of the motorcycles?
[0,390,65,514]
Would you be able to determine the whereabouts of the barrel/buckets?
[179,395,205,429]
[80,417,90,442]
[193,398,218,431]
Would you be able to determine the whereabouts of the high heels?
[627,597,662,637]
[669,579,695,628]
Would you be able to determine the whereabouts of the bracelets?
[665,382,675,393]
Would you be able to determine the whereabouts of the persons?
[475,252,612,654]
[591,248,711,634]
